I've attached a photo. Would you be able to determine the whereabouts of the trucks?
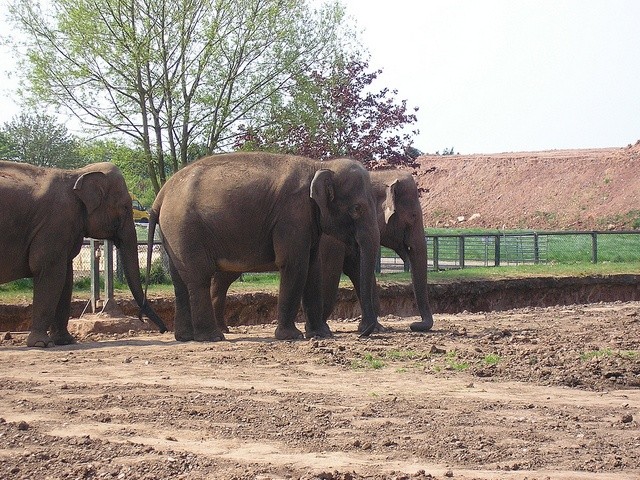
[128,192,150,223]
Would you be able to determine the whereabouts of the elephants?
[1,159,170,348]
[137,151,383,344]
[208,168,434,335]
